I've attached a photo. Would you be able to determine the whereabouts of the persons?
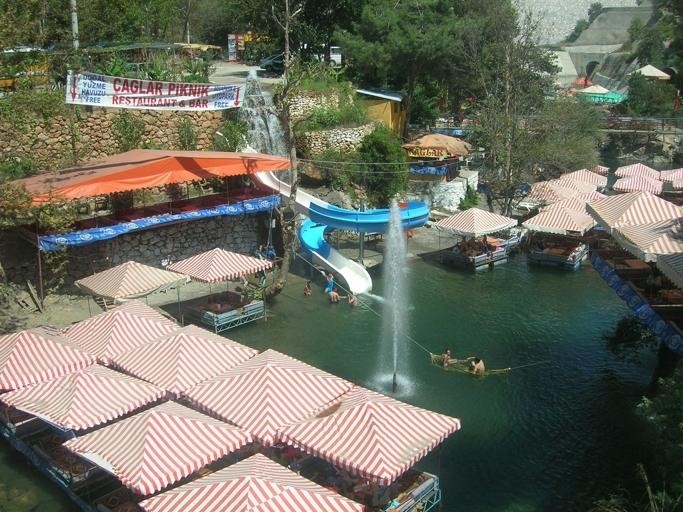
[439,348,461,370]
[301,280,313,297]
[463,357,486,376]
[327,286,342,304]
[439,235,587,259]
[324,273,333,294]
[346,290,357,309]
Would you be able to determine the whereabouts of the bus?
[0,47,50,94]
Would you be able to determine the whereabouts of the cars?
[257,44,342,73]
